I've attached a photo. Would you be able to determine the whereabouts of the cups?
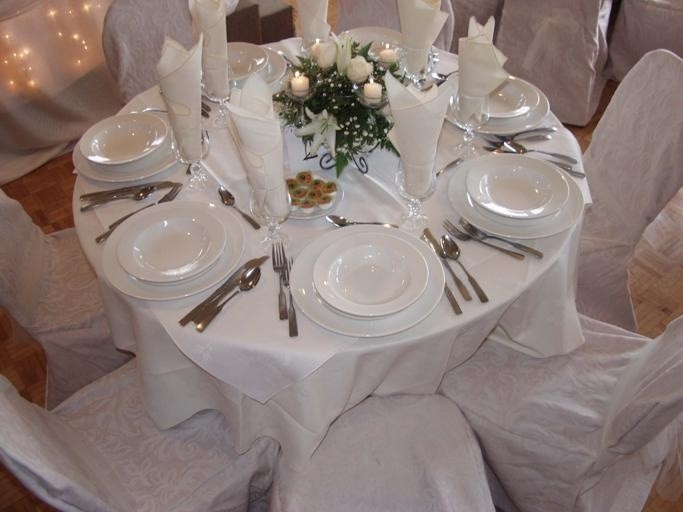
[395,159,439,218]
[397,45,433,88]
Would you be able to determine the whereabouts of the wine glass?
[249,177,292,249]
[449,91,491,162]
[169,128,214,194]
[201,67,236,129]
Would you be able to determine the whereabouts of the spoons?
[504,141,579,165]
[439,235,489,303]
[325,215,403,229]
[80,187,154,211]
[217,185,263,231]
[196,267,261,328]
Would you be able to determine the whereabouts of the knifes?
[422,228,472,300]
[192,256,270,323]
[80,182,176,200]
[178,257,259,327]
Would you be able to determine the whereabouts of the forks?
[95,184,183,244]
[271,242,288,321]
[282,256,299,338]
[494,127,557,140]
[442,219,526,260]
[457,218,544,260]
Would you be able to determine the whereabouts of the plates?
[289,223,445,340]
[338,25,403,44]
[438,73,551,135]
[227,39,288,95]
[71,110,180,182]
[101,199,247,302]
[447,154,587,239]
[287,171,342,219]
